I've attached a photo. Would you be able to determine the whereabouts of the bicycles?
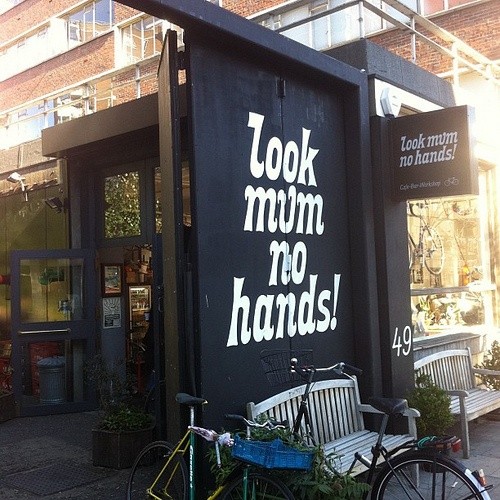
[407,201,445,275]
[286,357,494,500]
[125,393,312,500]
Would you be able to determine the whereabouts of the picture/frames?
[101,263,123,297]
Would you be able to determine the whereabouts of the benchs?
[246,375,421,489]
[413,347,500,459]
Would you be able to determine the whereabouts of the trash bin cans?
[36,355,66,405]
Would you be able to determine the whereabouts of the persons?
[129,314,162,406]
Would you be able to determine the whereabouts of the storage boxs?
[231,435,311,469]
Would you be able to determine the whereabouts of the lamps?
[7,172,25,192]
[44,197,63,214]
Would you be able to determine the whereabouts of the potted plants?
[473,340,500,420]
[91,400,156,470]
[0,386,15,424]
[402,372,456,474]
